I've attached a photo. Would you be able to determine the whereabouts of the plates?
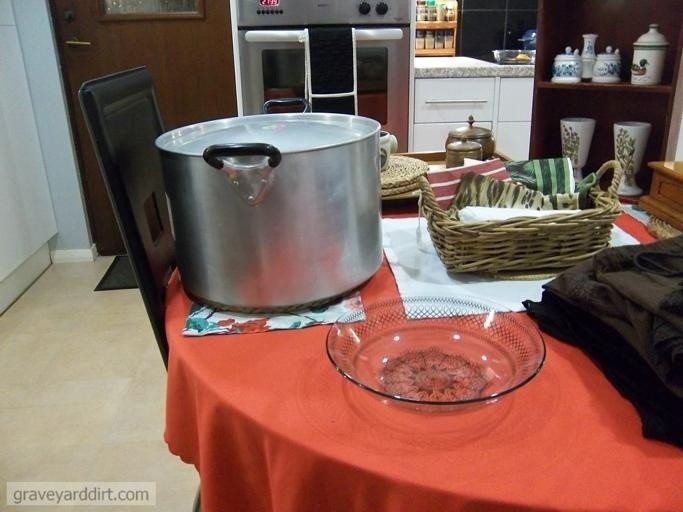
[326,293,548,418]
[490,48,536,65]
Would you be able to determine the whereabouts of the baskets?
[415,158,625,274]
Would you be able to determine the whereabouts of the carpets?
[94,256,138,291]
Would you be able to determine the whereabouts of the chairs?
[77,65,172,373]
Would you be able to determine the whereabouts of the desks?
[162,195,682,511]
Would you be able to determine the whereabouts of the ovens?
[234,0,410,153]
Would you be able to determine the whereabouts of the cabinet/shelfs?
[414,77,535,162]
[528,0,683,207]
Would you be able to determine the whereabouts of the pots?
[151,95,384,317]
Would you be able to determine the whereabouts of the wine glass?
[609,117,653,202]
[556,114,597,187]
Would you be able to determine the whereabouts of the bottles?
[628,22,670,87]
[443,136,483,169]
[415,0,454,50]
[579,32,599,80]
[550,47,582,85]
[444,114,497,161]
[590,45,623,84]
